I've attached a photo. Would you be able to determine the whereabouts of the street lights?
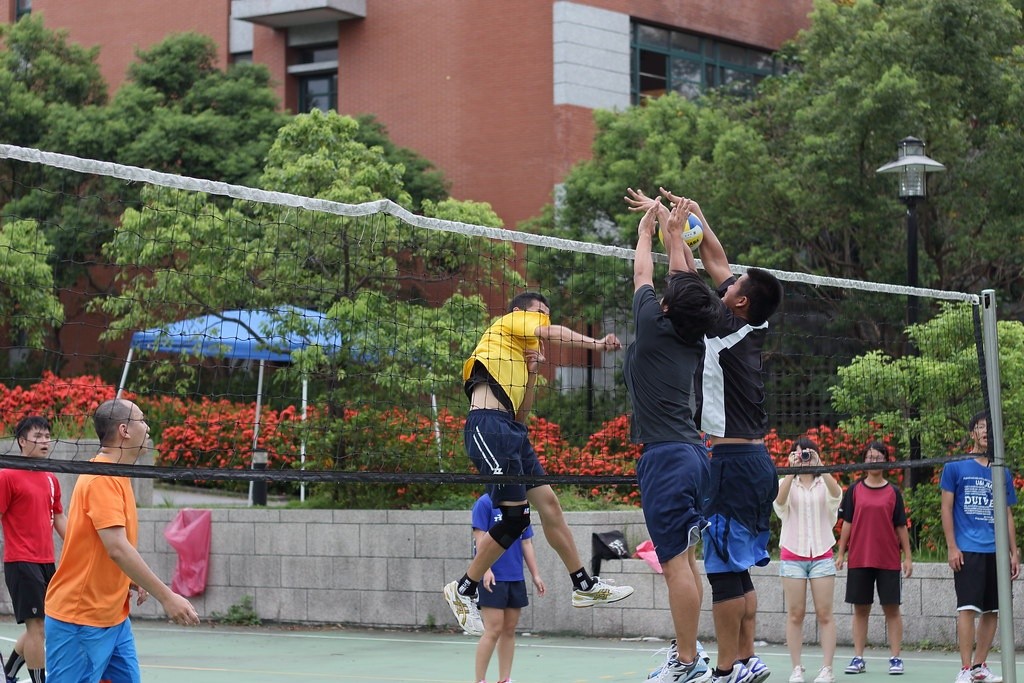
[876,135,949,553]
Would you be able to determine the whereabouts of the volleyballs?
[658,209,703,252]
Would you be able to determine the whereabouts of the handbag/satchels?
[593,530,629,560]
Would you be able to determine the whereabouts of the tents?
[115,305,442,509]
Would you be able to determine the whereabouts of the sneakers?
[712,660,755,683]
[971,663,1002,683]
[813,666,836,683]
[955,665,972,683]
[888,657,904,673]
[572,576,634,608]
[845,657,866,673]
[739,656,770,683]
[789,665,805,683]
[646,652,712,683]
[443,580,486,636]
[648,639,710,678]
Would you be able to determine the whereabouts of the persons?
[940,409,1020,683]
[471,493,546,683]
[0,416,68,683]
[834,442,913,674]
[446,293,635,637]
[626,186,783,683]
[773,437,843,683]
[43,399,200,683]
